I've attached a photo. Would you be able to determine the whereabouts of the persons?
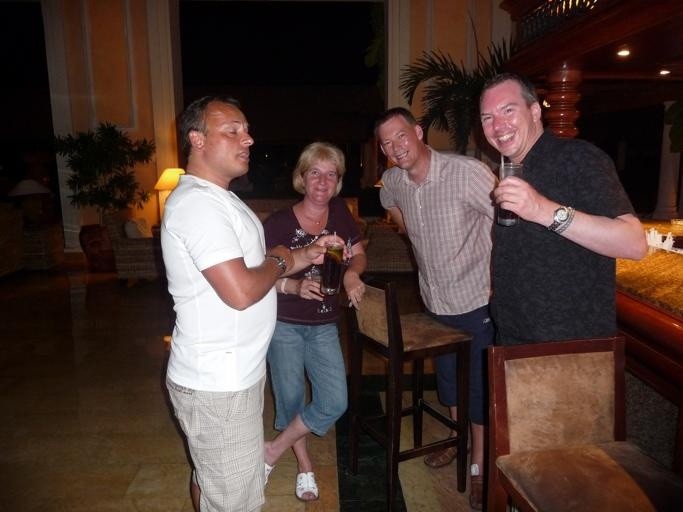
[263,143,366,502]
[380,107,500,510]
[159,97,352,512]
[484,73,632,346]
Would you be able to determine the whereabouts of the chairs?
[111,238,159,287]
[342,269,472,493]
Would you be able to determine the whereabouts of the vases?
[79,224,115,273]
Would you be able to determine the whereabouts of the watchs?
[546,205,569,233]
[265,254,285,276]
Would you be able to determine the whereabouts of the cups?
[321,242,343,296]
[497,163,523,226]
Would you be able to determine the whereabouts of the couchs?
[104,206,167,288]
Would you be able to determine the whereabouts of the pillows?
[125,215,153,238]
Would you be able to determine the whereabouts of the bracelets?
[553,209,576,235]
[280,279,289,295]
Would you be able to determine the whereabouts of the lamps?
[154,168,187,190]
[8,176,51,231]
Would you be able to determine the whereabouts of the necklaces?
[302,208,328,225]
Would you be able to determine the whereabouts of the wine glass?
[311,267,335,314]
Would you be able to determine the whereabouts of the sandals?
[294,467,321,503]
[467,468,485,510]
[263,461,275,488]
[423,433,471,468]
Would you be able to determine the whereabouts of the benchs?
[485,338,683,512]
